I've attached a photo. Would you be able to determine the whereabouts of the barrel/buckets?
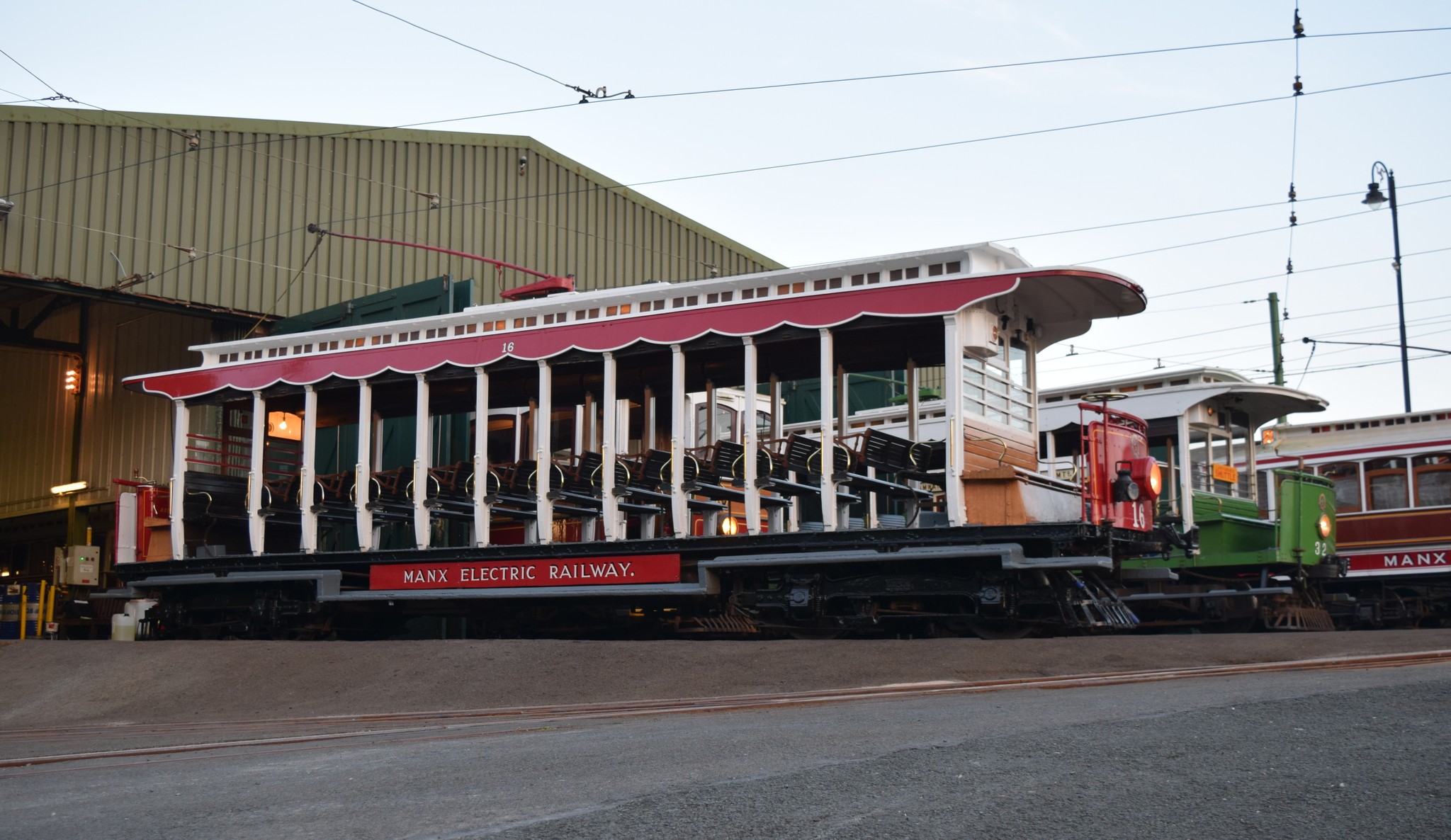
[111,613,135,641]
[125,599,158,635]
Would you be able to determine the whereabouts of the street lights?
[1362,161,1412,413]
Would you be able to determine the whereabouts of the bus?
[75,223,1451,634]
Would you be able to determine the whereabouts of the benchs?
[183,429,946,552]
